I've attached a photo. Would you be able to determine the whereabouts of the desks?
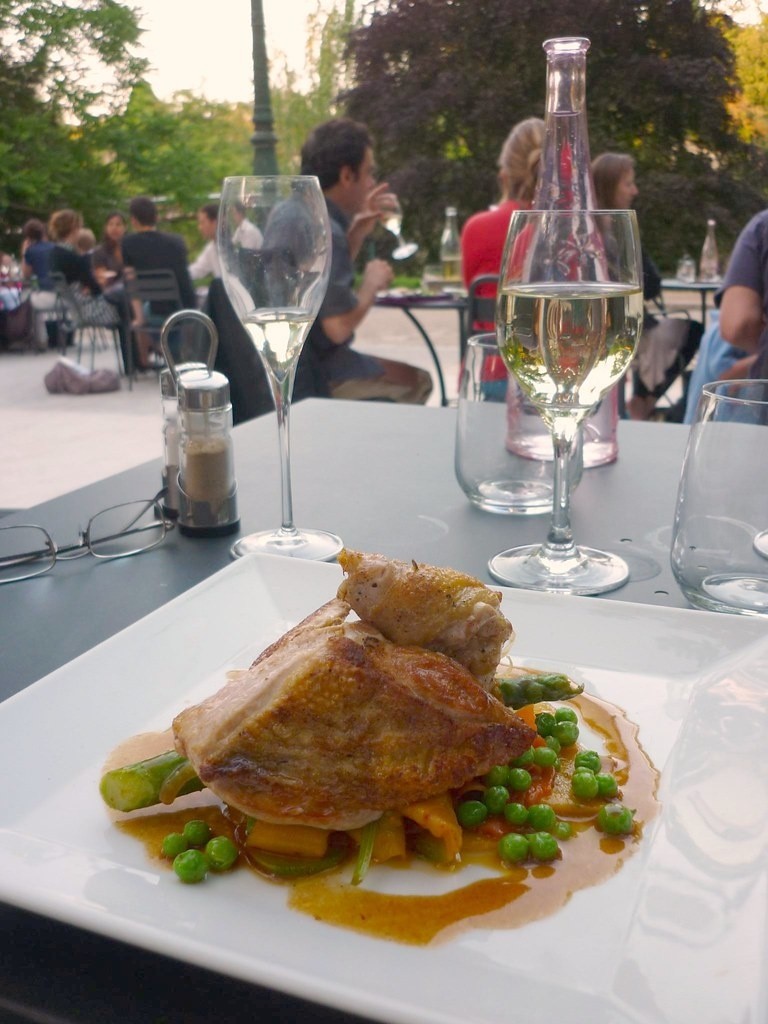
[0,280,768,1024]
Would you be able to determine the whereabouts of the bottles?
[160,362,207,522]
[440,206,461,282]
[506,37,618,470]
[700,219,719,278]
[177,368,241,537]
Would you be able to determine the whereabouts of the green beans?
[161,834,187,857]
[173,849,207,882]
[205,836,237,869]
[457,673,632,863]
[184,821,210,844]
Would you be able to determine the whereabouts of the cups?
[422,265,444,294]
[670,379,768,617]
[216,175,341,561]
[455,332,583,516]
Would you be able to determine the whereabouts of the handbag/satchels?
[0,300,33,341]
[45,318,74,346]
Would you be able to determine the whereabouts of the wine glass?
[488,210,643,596]
[374,192,419,260]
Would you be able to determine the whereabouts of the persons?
[260,119,435,406]
[186,206,230,286]
[261,171,327,269]
[462,117,600,405]
[584,152,661,421]
[226,199,266,252]
[122,196,202,368]
[684,208,767,425]
[0,206,150,373]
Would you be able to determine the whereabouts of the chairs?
[55,288,133,392]
[464,274,506,401]
[52,272,108,356]
[123,270,195,390]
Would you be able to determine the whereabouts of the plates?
[0,553,768,1024]
